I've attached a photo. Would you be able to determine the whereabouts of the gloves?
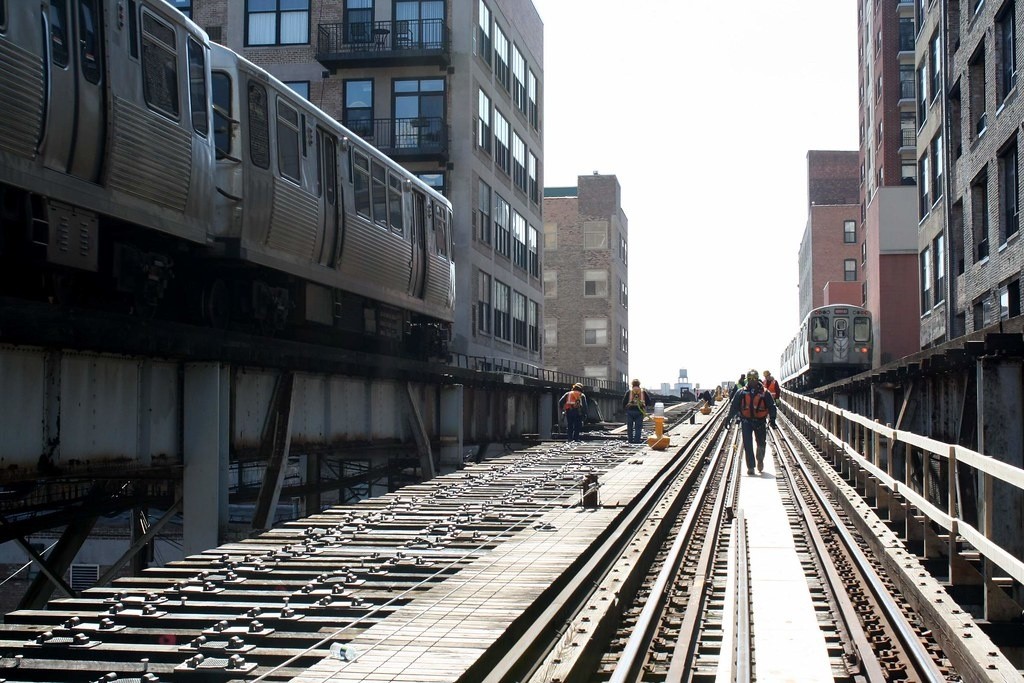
[769,418,775,427]
[725,419,731,430]
[776,399,781,405]
[584,412,588,419]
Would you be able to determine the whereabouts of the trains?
[0,1,456,358]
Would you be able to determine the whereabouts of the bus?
[777,302,875,390]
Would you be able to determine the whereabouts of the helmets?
[575,383,583,389]
[763,371,770,376]
[632,379,640,385]
[747,369,759,378]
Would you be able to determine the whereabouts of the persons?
[762,370,781,428]
[558,383,589,443]
[622,379,652,443]
[728,373,747,428]
[724,369,776,475]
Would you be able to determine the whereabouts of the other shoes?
[758,464,763,472]
[629,440,642,443]
[747,469,755,475]
[568,438,581,442]
